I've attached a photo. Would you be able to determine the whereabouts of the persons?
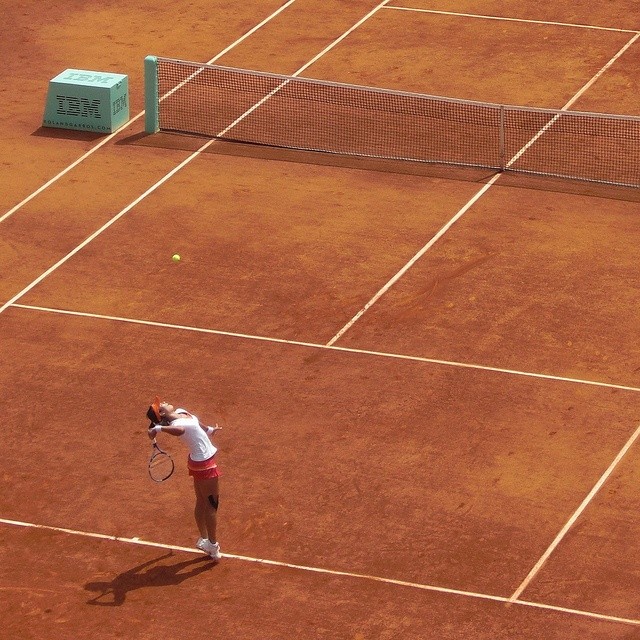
[146,396,222,560]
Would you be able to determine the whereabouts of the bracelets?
[155,425,161,433]
[206,426,213,435]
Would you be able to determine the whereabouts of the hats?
[151,395,161,423]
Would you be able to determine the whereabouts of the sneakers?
[195,537,209,552]
[202,540,222,561]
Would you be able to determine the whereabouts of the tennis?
[172,254,181,264]
